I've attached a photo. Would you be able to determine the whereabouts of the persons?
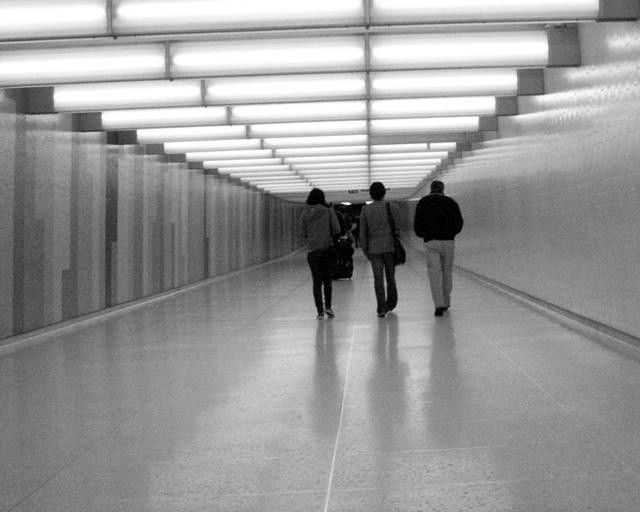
[299,188,341,319]
[359,181,402,317]
[413,180,464,316]
[325,201,361,282]
[308,315,344,439]
[369,313,407,427]
[425,309,462,431]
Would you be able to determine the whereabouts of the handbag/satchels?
[330,247,353,281]
[394,239,406,266]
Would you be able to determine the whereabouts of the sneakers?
[378,301,396,318]
[434,305,450,317]
[317,309,334,319]
